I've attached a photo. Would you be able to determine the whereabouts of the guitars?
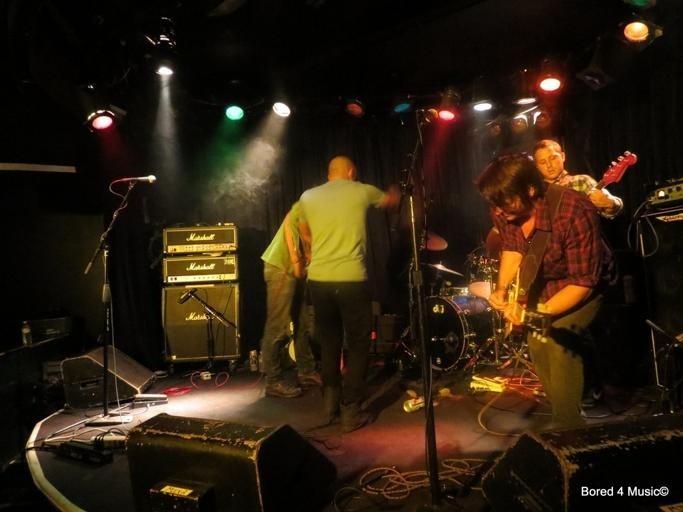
[503,283,550,344]
[586,149,636,201]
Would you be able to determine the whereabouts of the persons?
[298,155,403,435]
[259,201,323,398]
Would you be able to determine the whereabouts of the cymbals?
[426,262,464,277]
[420,231,448,250]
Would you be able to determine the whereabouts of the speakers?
[124,412,337,512]
[640,205,683,393]
[60,344,158,408]
[478,413,683,511]
[161,284,242,362]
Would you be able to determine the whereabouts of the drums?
[468,255,500,300]
[418,294,500,372]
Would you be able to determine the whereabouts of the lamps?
[436,12,664,122]
[344,95,369,120]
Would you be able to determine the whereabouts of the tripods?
[461,267,535,374]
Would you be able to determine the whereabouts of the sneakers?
[298,371,324,385]
[264,379,302,398]
[339,401,376,433]
[322,386,342,419]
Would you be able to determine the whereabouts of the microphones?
[417,108,439,123]
[445,336,456,346]
[112,174,157,184]
[176,287,198,305]
